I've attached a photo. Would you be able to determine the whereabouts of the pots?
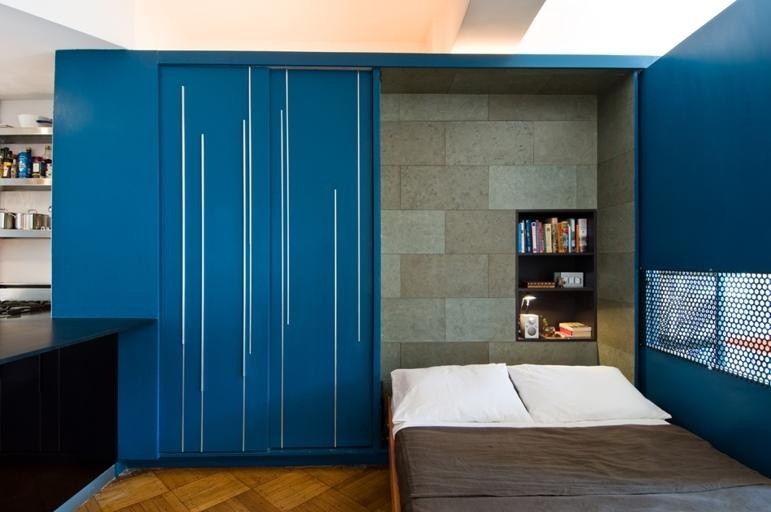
[0,208,51,230]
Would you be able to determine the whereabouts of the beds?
[383,359,770,511]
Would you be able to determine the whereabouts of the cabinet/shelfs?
[51,48,387,468]
[516,208,598,342]
[0,125,53,239]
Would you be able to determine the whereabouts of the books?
[559,327,591,337]
[515,215,588,254]
[559,321,591,331]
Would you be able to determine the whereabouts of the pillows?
[387,361,674,426]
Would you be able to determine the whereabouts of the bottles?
[0,145,53,179]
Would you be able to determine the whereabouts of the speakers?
[519,314,540,339]
